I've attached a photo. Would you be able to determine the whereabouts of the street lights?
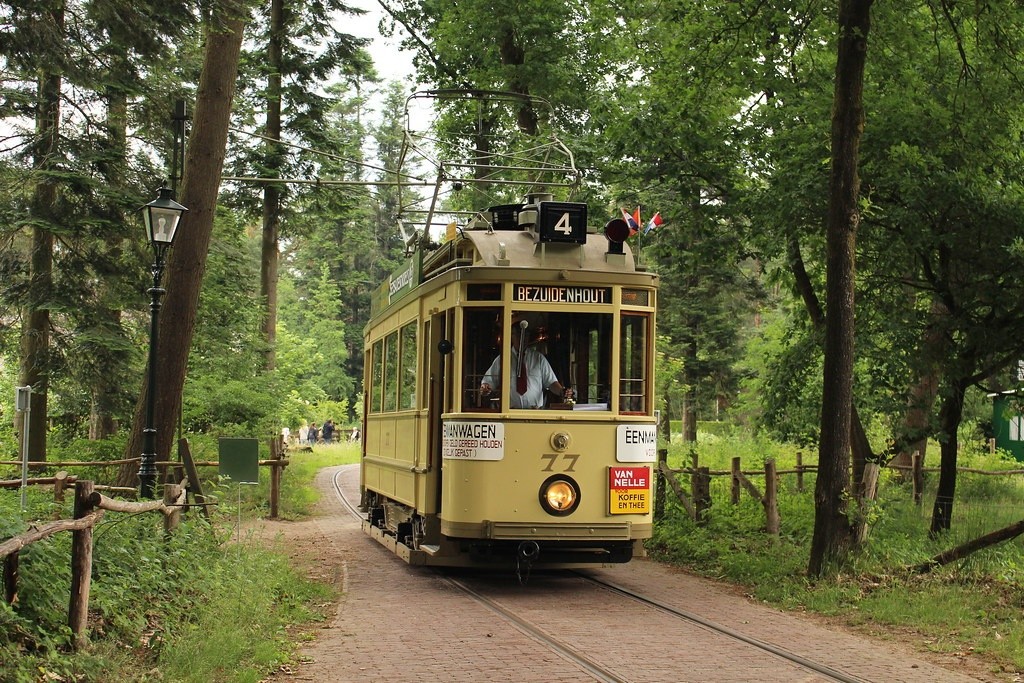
[136,178,189,500]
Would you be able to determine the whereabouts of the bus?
[358,88,660,568]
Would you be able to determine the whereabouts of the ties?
[516,353,527,396]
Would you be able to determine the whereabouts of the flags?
[620,205,663,236]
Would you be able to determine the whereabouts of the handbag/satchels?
[355,431,360,438]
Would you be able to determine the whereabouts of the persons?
[299,420,336,445]
[480,321,573,409]
[351,427,361,442]
